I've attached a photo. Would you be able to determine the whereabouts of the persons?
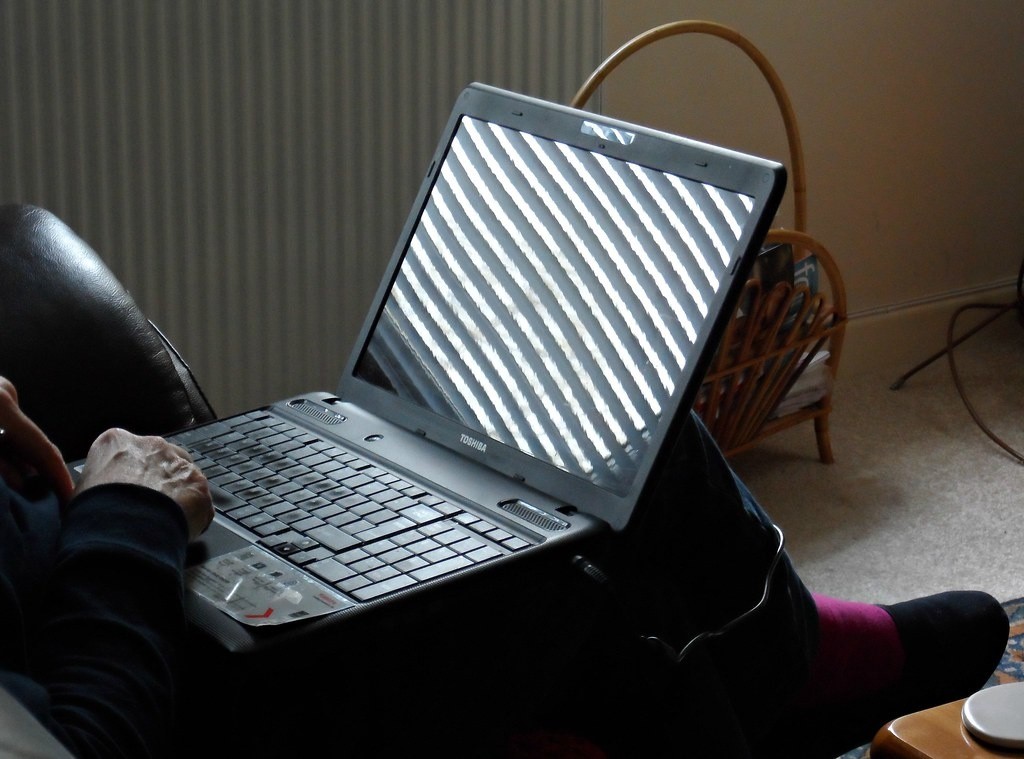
[1,373,1010,759]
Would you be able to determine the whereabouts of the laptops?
[69,81,788,659]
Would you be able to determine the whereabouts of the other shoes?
[778,593,1010,759]
